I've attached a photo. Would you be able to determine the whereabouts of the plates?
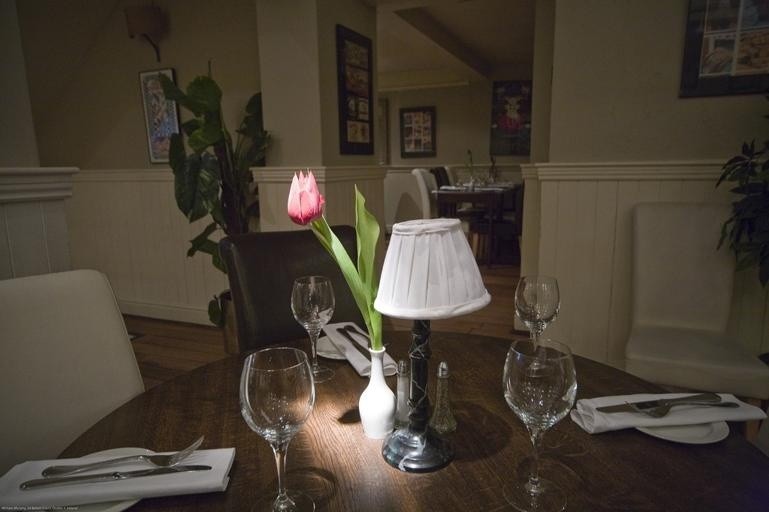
[634,421,731,445]
[67,498,142,512]
[316,334,349,361]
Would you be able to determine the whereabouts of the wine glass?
[238,346,317,512]
[513,274,560,378]
[291,275,336,384]
[502,339,581,512]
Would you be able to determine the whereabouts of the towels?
[0,448,235,508]
[322,322,397,377]
[571,392,768,434]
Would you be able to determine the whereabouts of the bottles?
[394,357,413,430]
[430,361,459,435]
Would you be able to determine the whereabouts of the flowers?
[286,170,384,349]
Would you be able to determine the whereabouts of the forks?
[344,325,391,348]
[41,434,205,478]
[626,402,740,418]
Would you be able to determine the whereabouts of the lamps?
[373,218,491,473]
[125,5,160,63]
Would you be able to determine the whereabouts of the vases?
[359,349,397,439]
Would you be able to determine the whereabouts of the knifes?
[20,464,212,491]
[336,328,371,360]
[596,391,722,414]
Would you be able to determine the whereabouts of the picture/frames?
[338,24,436,164]
[138,69,181,164]
[491,81,530,155]
[678,0,769,99]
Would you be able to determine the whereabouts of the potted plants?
[156,71,273,354]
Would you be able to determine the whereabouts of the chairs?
[1,270,147,474]
[413,169,468,233]
[220,227,364,352]
[431,166,483,220]
[626,201,769,444]
[470,183,523,264]
[445,165,481,211]
[753,409,768,458]
[491,183,520,216]
